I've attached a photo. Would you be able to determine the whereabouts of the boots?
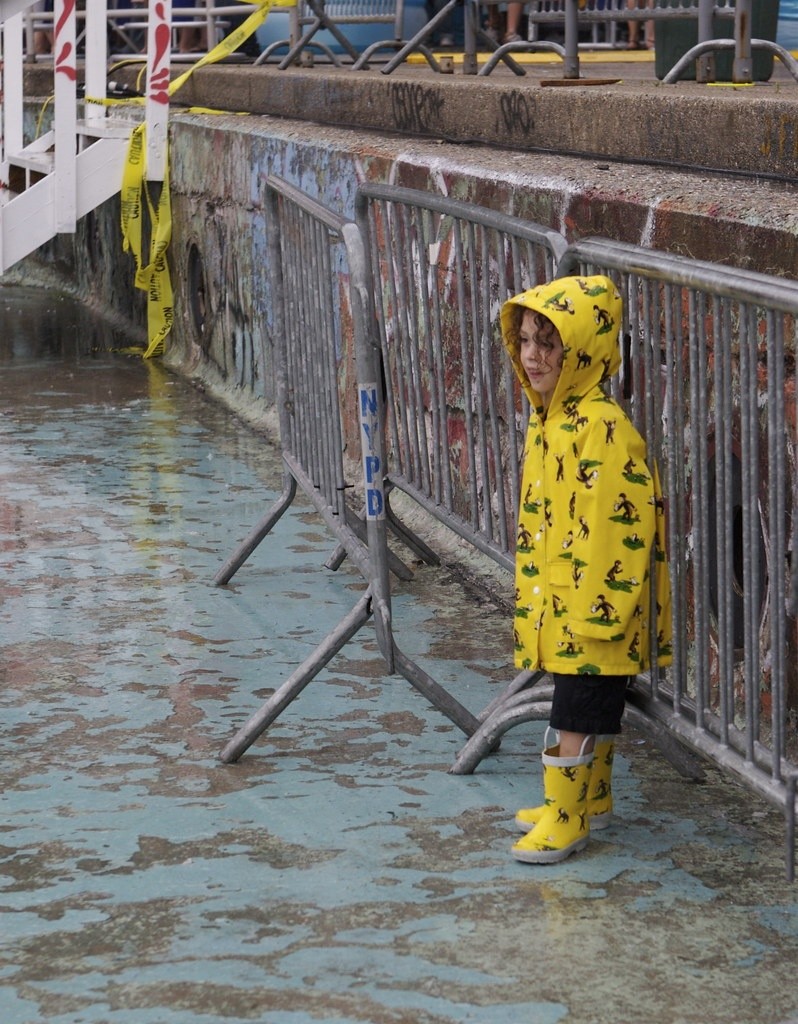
[511,726,594,865]
[515,733,615,829]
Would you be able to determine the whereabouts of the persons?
[500,275,673,864]
